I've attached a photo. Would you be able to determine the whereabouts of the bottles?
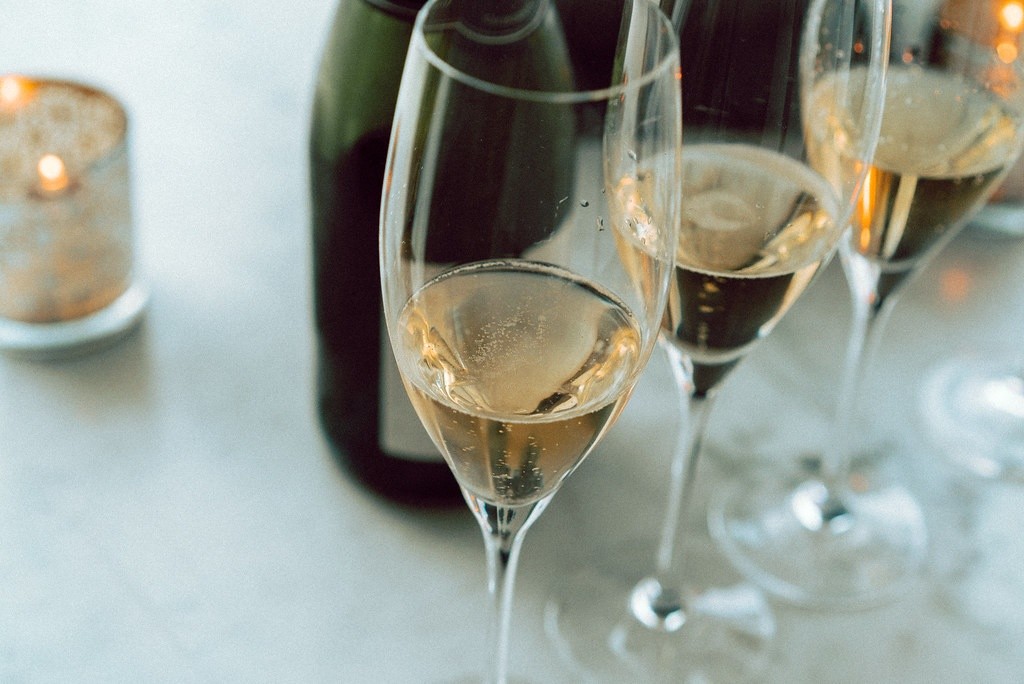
[307,4,587,515]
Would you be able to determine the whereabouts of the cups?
[0,64,153,354]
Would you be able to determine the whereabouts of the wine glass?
[542,2,896,684]
[705,0,1023,609]
[378,3,684,684]
